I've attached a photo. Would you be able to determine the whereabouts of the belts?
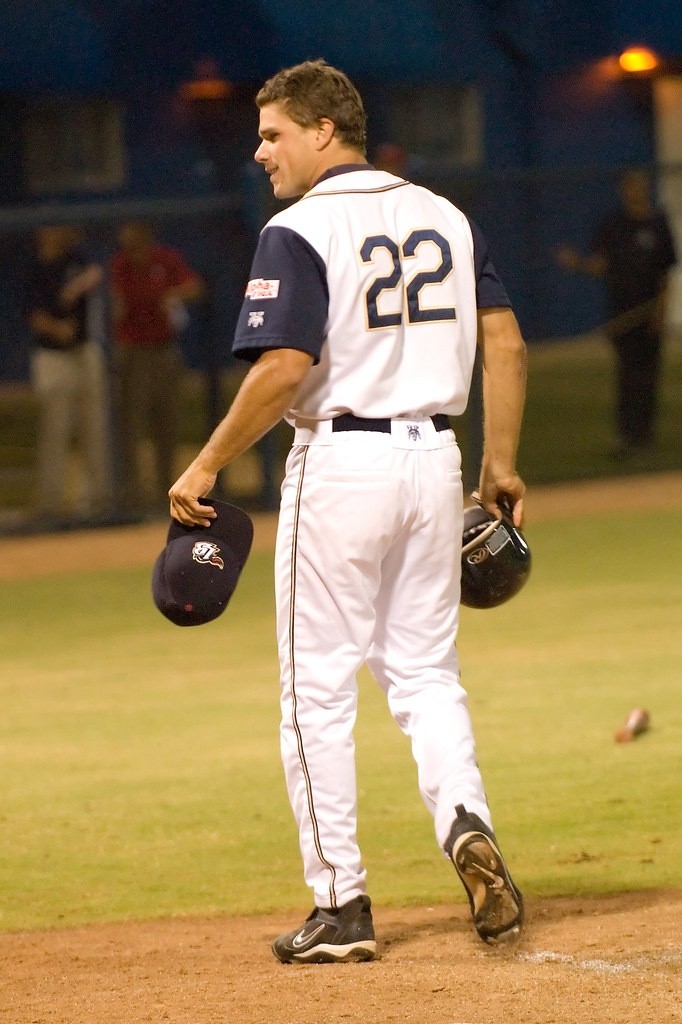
[330,412,452,434]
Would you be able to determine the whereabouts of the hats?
[151,497,254,627]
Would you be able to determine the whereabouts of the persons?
[170,61,529,962]
[556,165,679,458]
[18,139,419,529]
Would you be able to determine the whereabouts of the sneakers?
[269,894,377,965]
[443,803,524,945]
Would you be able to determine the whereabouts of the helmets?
[460,488,531,610]
